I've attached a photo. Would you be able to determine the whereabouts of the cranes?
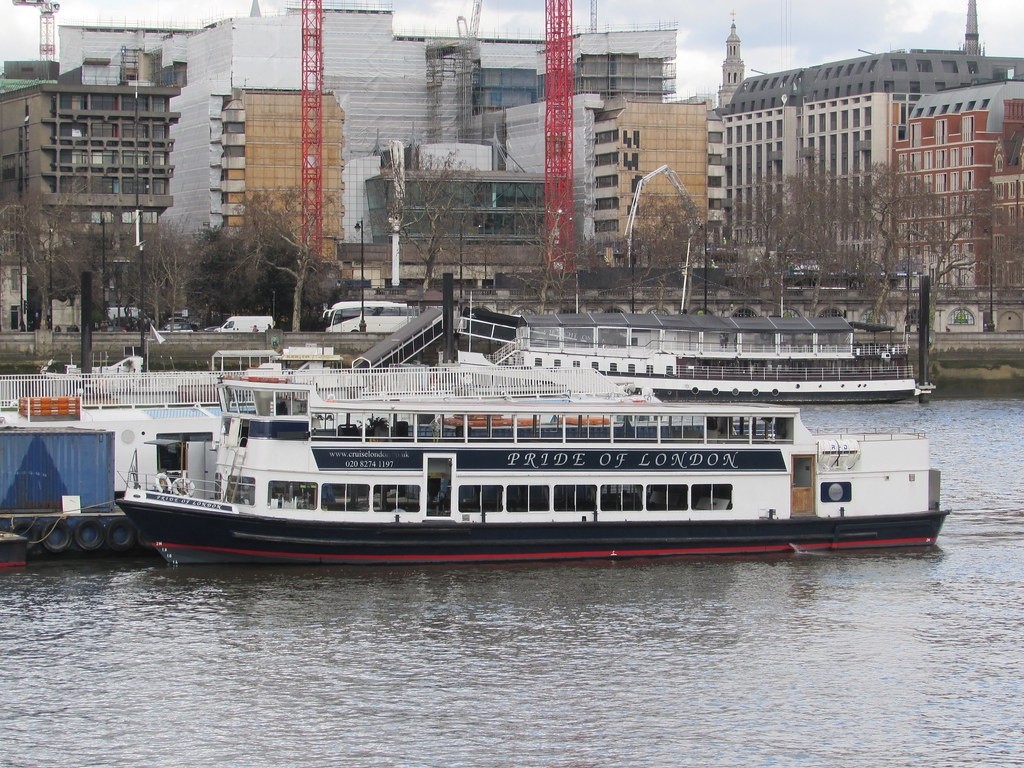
[12,0,59,61]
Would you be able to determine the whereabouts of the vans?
[214,316,274,332]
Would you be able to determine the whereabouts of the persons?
[73,325,79,332]
[268,324,271,329]
[55,325,61,332]
[253,325,259,332]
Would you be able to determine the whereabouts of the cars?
[160,322,192,330]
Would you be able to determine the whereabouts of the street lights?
[353,220,366,332]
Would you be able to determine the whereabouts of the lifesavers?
[170,476,196,497]
[9,519,40,552]
[105,518,139,553]
[74,516,106,551]
[155,472,172,493]
[41,518,74,554]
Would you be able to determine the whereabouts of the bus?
[323,301,443,332]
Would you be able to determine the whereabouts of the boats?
[458,314,915,403]
[115,366,952,564]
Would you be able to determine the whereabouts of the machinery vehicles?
[622,165,702,313]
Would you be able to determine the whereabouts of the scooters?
[41,356,77,375]
